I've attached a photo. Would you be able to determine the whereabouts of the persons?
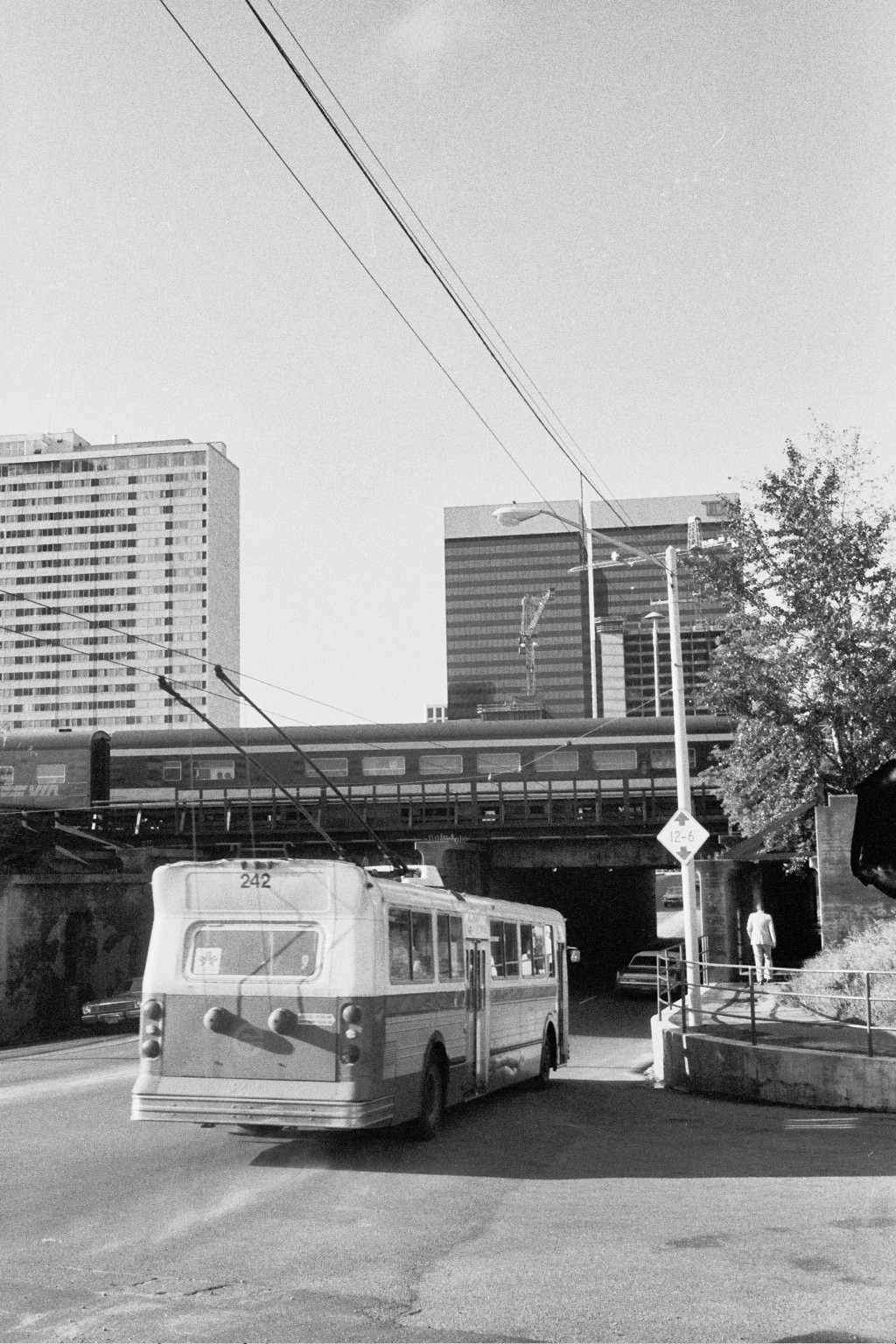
[490,956,497,976]
[747,902,777,985]
[226,773,232,778]
[217,772,224,780]
[527,925,553,977]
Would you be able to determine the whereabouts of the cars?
[618,950,687,999]
[80,976,143,1036]
[662,886,684,907]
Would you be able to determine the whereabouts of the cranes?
[570,516,731,628]
[516,586,558,699]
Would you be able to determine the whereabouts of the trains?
[0,713,741,817]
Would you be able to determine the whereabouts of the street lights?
[494,506,704,1030]
[643,610,666,717]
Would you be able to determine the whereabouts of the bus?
[128,856,572,1142]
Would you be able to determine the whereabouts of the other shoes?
[767,979,772,983]
[759,981,763,985]
